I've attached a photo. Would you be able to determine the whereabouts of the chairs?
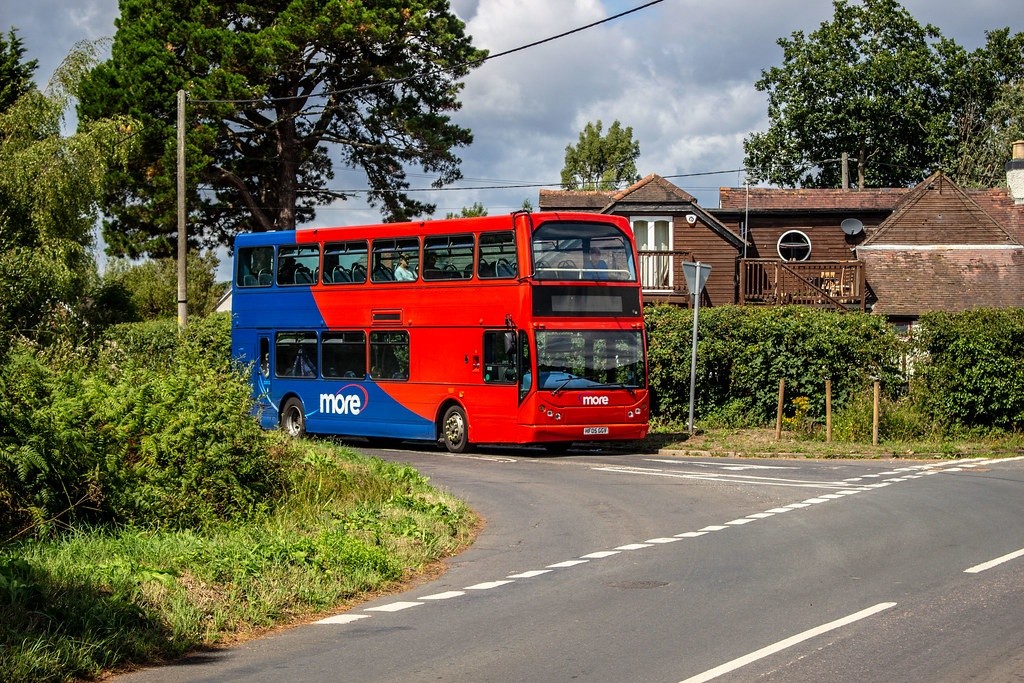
[242,258,582,285]
[285,366,406,380]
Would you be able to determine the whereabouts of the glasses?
[592,251,600,254]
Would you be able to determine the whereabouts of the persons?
[393,253,417,281]
[584,247,608,280]
[280,258,303,274]
[501,345,528,375]
[426,252,442,279]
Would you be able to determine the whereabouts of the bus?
[230,208,655,453]
